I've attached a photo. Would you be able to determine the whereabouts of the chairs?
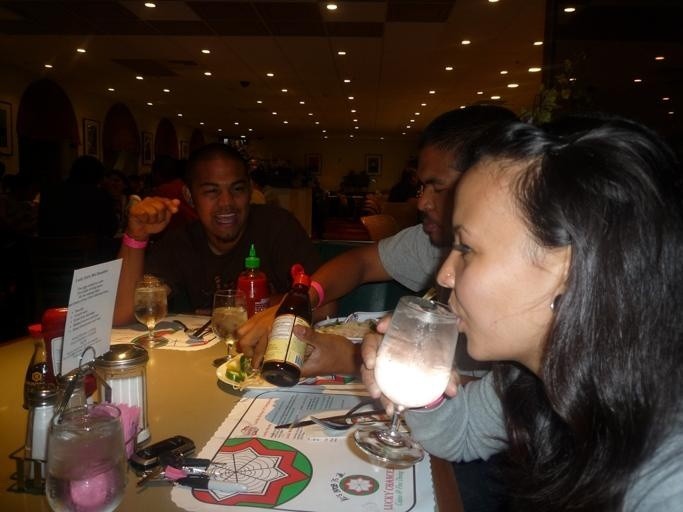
[311,241,435,317]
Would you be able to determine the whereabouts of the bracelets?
[309,281,326,310]
[123,234,148,249]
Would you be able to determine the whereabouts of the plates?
[315,310,383,343]
[218,354,366,395]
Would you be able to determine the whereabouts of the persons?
[360,117,683,512]
[2,145,198,341]
[236,105,530,377]
[113,143,338,327]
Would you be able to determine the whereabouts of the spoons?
[311,399,371,431]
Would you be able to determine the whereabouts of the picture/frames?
[180,140,189,160]
[83,117,98,158]
[365,154,382,176]
[304,153,322,177]
[0,100,13,155]
[142,131,153,166]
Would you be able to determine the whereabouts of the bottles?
[94,342,148,446]
[25,323,86,488]
[261,264,312,386]
[234,244,269,317]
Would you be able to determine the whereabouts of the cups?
[46,404,128,510]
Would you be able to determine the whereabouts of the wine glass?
[134,270,171,348]
[354,296,461,468]
[212,289,241,366]
